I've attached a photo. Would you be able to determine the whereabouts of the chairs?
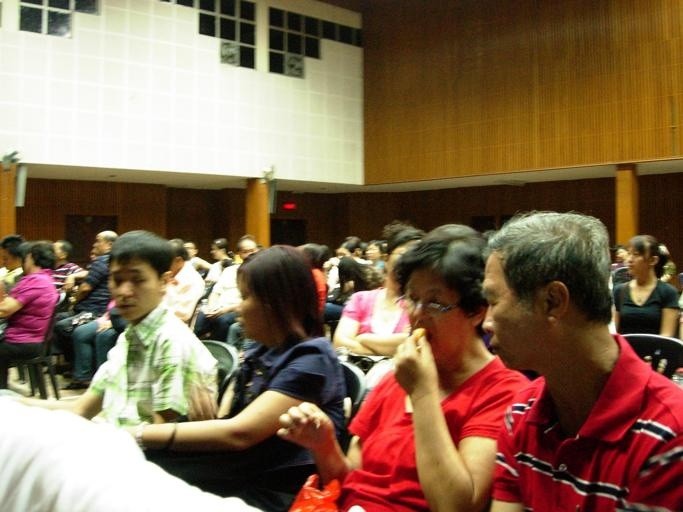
[200,339,240,407]
[610,266,637,292]
[620,333,683,388]
[0,288,67,400]
[340,361,368,419]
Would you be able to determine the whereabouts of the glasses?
[392,294,458,318]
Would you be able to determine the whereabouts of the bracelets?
[137,420,143,444]
[165,418,176,448]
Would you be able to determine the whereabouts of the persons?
[118,245,348,512]
[0,394,272,512]
[0,219,683,390]
[275,224,531,512]
[73,230,219,452]
[481,209,683,512]
